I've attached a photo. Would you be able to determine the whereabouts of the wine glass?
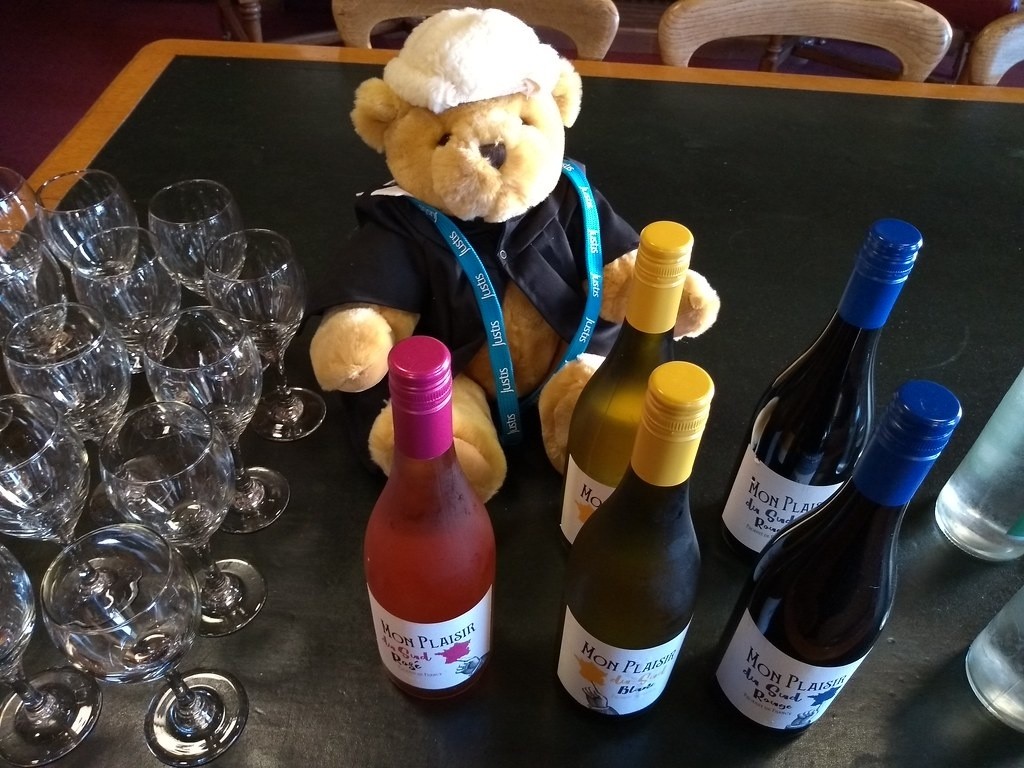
[72,225,195,417]
[207,228,328,439]
[142,305,290,533]
[3,301,173,521]
[0,394,141,629]
[43,524,250,768]
[35,167,178,369]
[0,545,103,768]
[102,403,266,639]
[0,168,73,350]
[146,179,245,380]
[0,231,106,412]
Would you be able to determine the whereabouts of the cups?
[935,368,1024,561]
[967,586,1024,735]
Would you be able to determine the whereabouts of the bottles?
[368,338,496,705]
[707,381,962,734]
[548,363,713,720]
[724,219,923,568]
[557,222,693,558]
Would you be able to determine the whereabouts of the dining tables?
[1,39,1022,766]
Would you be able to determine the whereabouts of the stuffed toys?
[309,9,721,504]
